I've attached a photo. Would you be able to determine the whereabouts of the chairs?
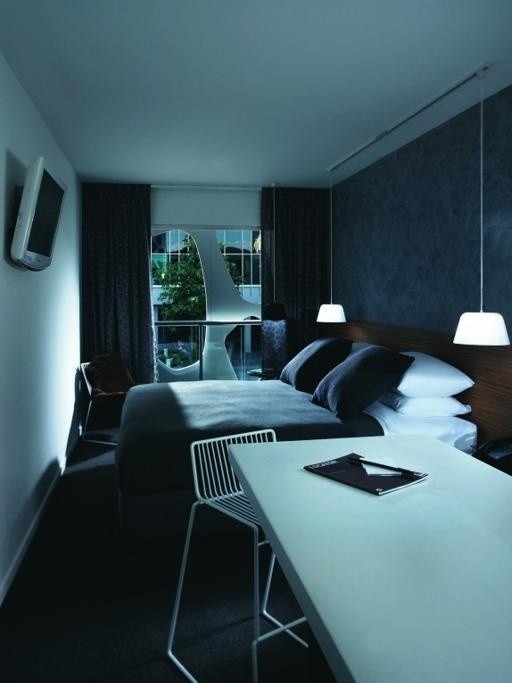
[81,362,134,446]
[247,320,286,380]
[167,429,309,683]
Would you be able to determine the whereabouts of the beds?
[112,320,475,496]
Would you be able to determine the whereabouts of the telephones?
[470,433,512,461]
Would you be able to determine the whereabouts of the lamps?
[452,61,510,346]
[316,167,347,322]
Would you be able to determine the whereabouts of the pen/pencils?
[347,456,413,476]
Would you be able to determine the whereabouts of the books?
[302,451,429,496]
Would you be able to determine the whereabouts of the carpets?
[0,432,339,683]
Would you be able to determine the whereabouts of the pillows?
[280,336,352,390]
[309,346,415,417]
[398,353,475,395]
[380,392,472,414]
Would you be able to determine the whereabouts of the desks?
[228,436,512,683]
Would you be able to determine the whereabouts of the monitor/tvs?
[8,156,69,273]
